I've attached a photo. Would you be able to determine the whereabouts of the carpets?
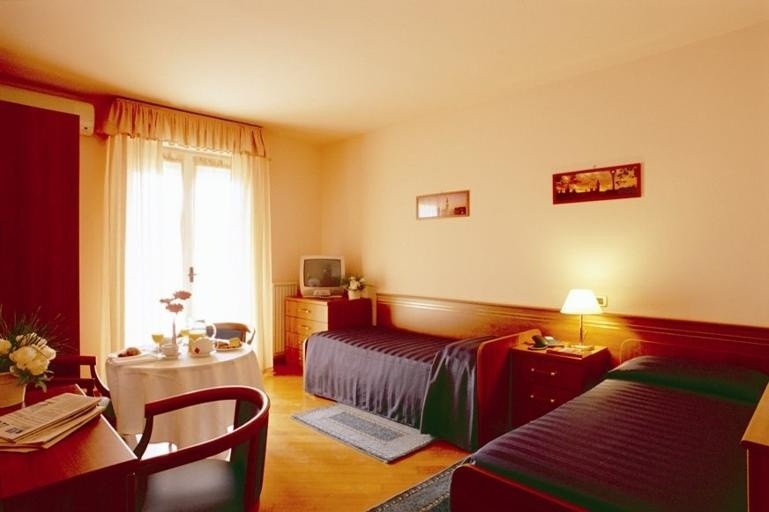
[291,400,439,465]
[366,457,465,511]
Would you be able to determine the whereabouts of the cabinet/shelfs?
[283,294,372,367]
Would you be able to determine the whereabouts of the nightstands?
[506,339,609,433]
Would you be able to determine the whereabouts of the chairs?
[132,384,271,512]
[206,322,256,346]
[26,355,115,430]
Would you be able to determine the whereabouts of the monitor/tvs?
[299,256,345,297]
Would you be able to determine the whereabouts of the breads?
[229,337,240,347]
[118,347,139,356]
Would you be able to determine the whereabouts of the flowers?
[0,304,78,393]
[159,290,193,315]
[342,272,368,291]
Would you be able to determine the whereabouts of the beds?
[449,354,769,512]
[302,324,543,453]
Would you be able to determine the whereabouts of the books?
[0,391,111,454]
[546,345,593,358]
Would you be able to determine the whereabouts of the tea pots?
[189,333,217,358]
[185,319,217,352]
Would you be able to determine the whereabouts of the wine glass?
[151,333,165,354]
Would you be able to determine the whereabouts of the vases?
[0,371,27,408]
[348,290,361,301]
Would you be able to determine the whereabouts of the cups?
[161,343,179,359]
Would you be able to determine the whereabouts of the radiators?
[272,281,298,357]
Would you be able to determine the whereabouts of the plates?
[215,345,241,351]
[107,349,148,361]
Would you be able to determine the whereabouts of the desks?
[0,383,139,511]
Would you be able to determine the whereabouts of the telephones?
[532,335,564,347]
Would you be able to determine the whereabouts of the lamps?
[560,288,603,351]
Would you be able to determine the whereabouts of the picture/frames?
[552,163,641,205]
[416,190,470,220]
